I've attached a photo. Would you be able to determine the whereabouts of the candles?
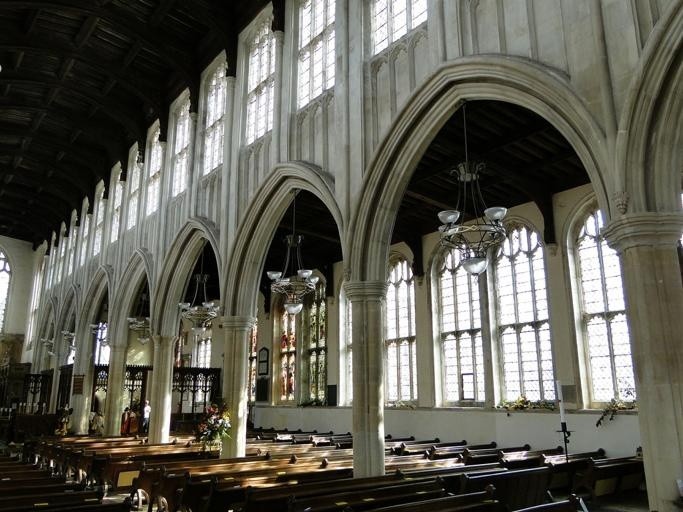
[556,380,566,423]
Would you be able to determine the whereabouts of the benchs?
[0,425,648,512]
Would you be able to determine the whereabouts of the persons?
[143,400,152,435]
[120,407,131,435]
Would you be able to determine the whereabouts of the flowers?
[595,397,637,428]
[191,396,232,457]
[502,394,557,417]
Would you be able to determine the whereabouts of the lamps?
[177,238,220,341]
[126,275,151,345]
[437,103,511,280]
[265,186,321,320]
